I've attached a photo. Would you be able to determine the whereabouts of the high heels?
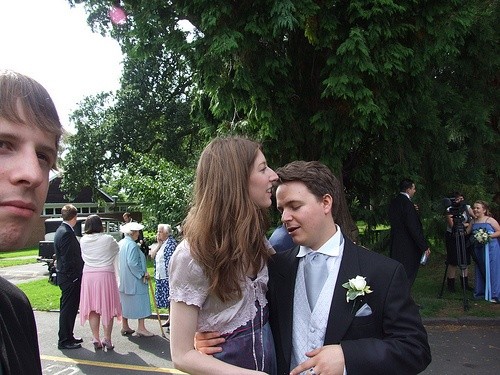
[136,329,154,337]
[101,339,114,352]
[121,328,135,335]
[93,339,102,350]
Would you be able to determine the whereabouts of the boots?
[447,277,456,293]
[460,276,474,291]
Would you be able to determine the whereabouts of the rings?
[310,368,316,375]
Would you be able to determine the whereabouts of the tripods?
[439,225,485,311]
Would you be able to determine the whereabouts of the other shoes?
[58,343,81,350]
[74,338,83,343]
[161,321,170,327]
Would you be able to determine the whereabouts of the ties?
[304,253,331,314]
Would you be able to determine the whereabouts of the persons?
[122,212,143,246]
[462,199,500,304]
[170,136,280,375]
[116,223,155,338]
[54,204,83,349]
[1,68,61,374]
[149,224,178,327]
[80,214,121,351]
[388,178,432,288]
[192,158,432,375]
[446,191,477,291]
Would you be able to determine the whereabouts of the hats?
[119,222,145,233]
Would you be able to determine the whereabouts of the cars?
[42,216,124,245]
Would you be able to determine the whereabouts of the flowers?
[471,226,492,245]
[341,275,374,307]
